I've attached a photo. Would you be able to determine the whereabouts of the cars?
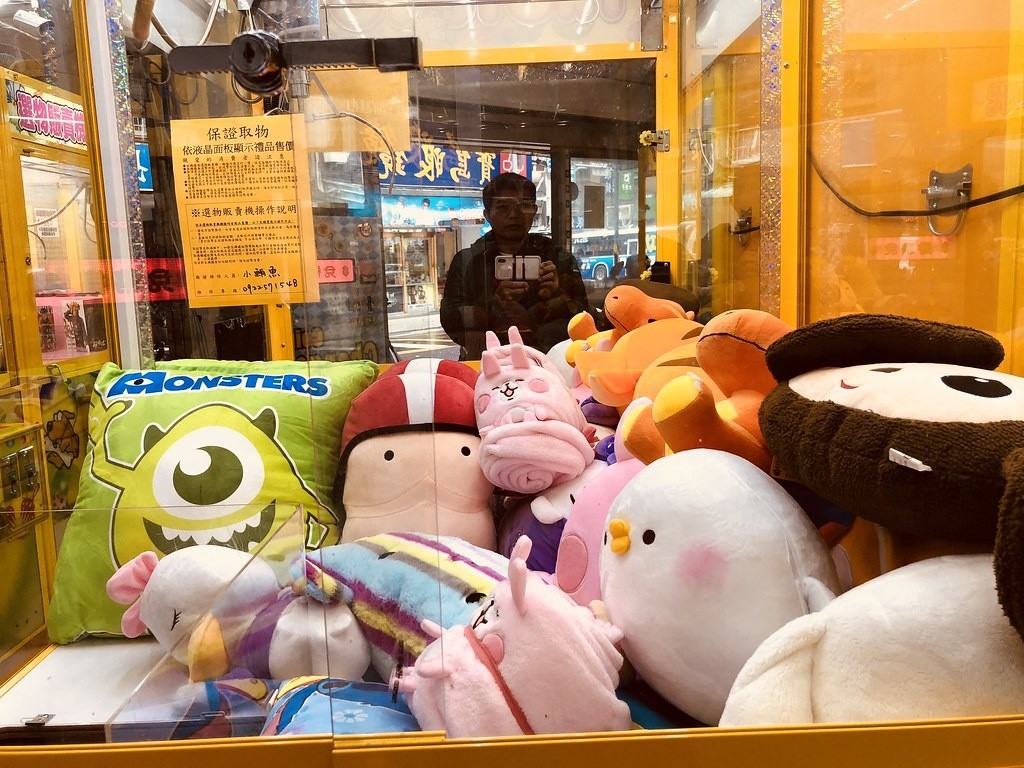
[385,264,410,282]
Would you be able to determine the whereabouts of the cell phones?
[495,256,541,280]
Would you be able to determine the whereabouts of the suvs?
[690,110,907,279]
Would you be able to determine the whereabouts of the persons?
[440,171,590,362]
[414,198,436,225]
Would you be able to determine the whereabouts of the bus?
[572,225,679,280]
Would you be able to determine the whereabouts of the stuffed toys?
[599,447,841,728]
[390,535,632,739]
[105,544,373,718]
[565,284,727,457]
[718,554,1024,727]
[758,314,1024,647]
[474,326,595,493]
[331,358,498,550]
[620,309,797,474]
